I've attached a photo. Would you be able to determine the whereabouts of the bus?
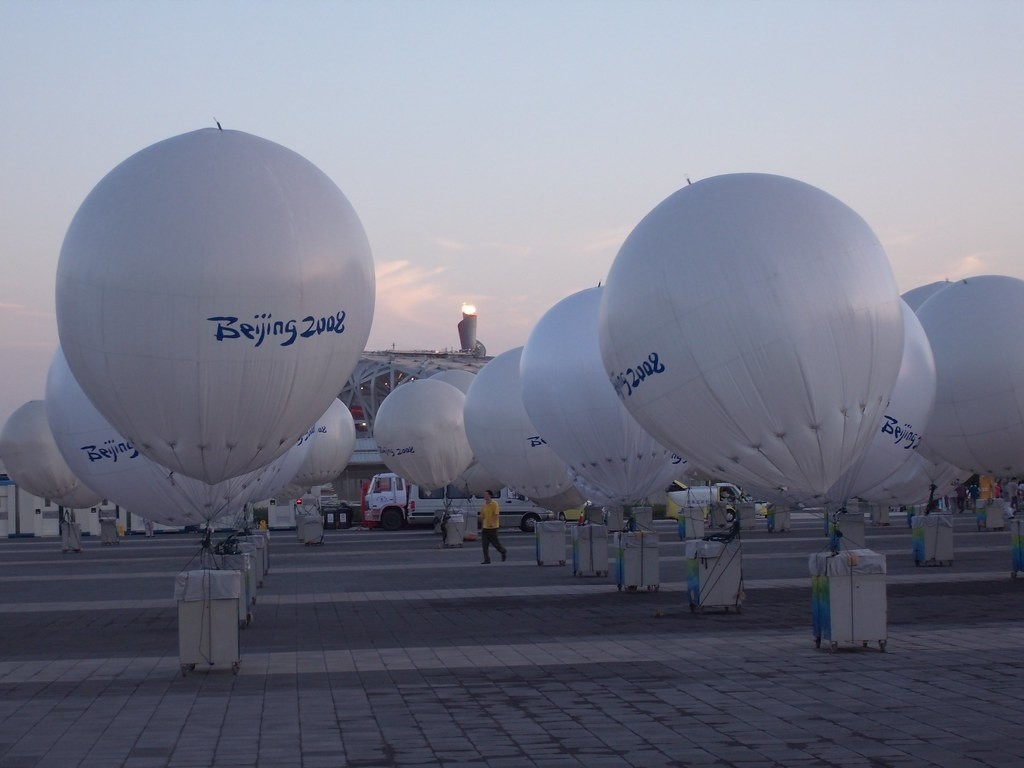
[406,478,554,533]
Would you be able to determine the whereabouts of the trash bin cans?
[323,508,337,530]
[338,508,352,529]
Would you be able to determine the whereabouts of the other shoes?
[481,561,490,565]
[502,548,507,561]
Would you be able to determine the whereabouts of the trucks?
[663,481,750,522]
[364,472,408,531]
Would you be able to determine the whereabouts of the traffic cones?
[578,511,585,525]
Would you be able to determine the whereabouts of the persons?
[478,490,507,565]
[995,477,1024,511]
[143,517,155,538]
[721,488,736,501]
[967,482,979,515]
[956,484,966,514]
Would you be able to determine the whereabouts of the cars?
[547,498,588,522]
